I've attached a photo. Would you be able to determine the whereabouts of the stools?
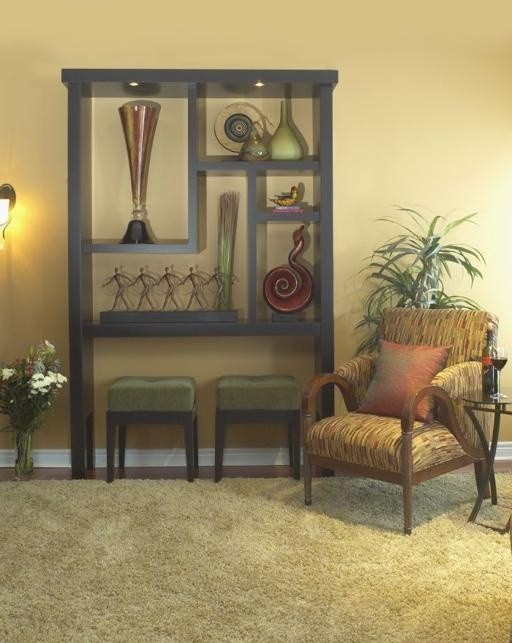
[105,375,303,484]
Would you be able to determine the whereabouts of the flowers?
[0,339,69,472]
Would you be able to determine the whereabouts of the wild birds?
[268,185,299,207]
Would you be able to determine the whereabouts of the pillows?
[357,339,453,426]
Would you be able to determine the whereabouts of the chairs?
[300,306,499,536]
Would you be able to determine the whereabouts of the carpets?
[0,472,512,643]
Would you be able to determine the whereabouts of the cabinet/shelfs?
[61,68,339,480]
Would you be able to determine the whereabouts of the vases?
[13,428,36,481]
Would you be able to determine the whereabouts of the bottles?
[480,319,501,401]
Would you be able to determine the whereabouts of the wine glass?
[490,345,509,401]
